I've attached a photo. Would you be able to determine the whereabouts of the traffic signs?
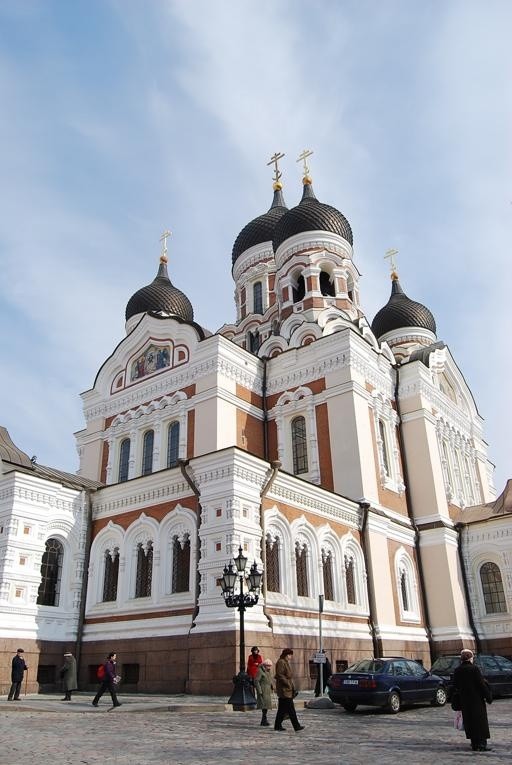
[316,654,326,658]
[314,657,327,663]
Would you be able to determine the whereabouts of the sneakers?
[61,697,72,701]
[260,720,270,727]
[471,745,492,752]
[7,696,22,701]
[274,723,306,732]
[92,700,122,707]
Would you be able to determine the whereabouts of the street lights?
[218,543,264,712]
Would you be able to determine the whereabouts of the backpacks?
[97,663,106,681]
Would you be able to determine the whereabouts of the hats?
[63,648,73,657]
[319,649,327,653]
[461,649,474,661]
[251,646,260,652]
[17,648,24,654]
[264,660,273,666]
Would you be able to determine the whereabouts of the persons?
[90,650,123,708]
[58,648,78,702]
[314,649,332,698]
[246,645,262,698]
[253,658,275,726]
[273,648,305,733]
[6,647,28,702]
[452,648,494,752]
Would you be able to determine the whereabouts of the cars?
[326,656,449,715]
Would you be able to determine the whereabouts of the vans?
[427,654,512,700]
